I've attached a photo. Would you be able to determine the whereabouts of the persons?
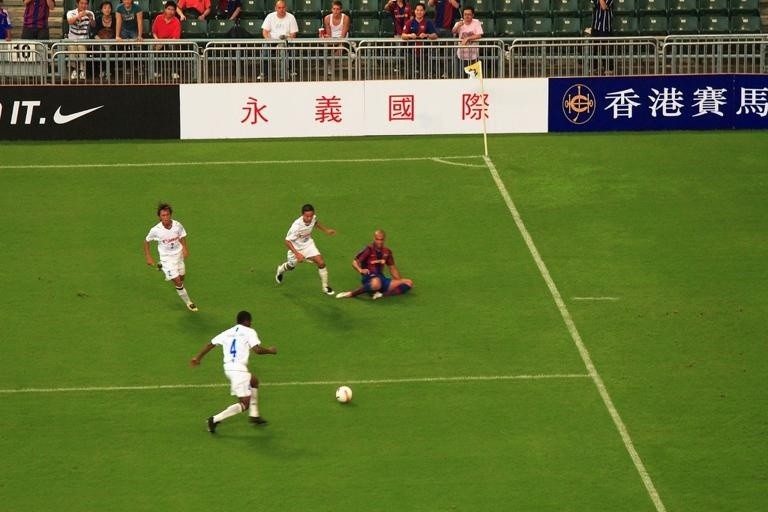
[21,1,56,41]
[274,203,337,295]
[114,0,145,75]
[90,1,116,79]
[401,3,438,77]
[427,0,461,39]
[256,0,299,80]
[585,0,616,76]
[323,1,350,77]
[336,228,413,302]
[66,1,97,80]
[450,6,483,79]
[144,203,199,313]
[0,1,13,41]
[187,309,278,435]
[383,1,413,39]
[175,0,212,23]
[150,1,182,79]
[214,1,243,22]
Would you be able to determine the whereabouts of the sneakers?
[289,70,297,76]
[335,291,352,298]
[588,68,598,73]
[256,73,268,80]
[154,72,161,77]
[79,70,86,80]
[322,286,335,296]
[206,416,220,434]
[71,70,78,80]
[604,70,613,75]
[372,291,383,301]
[275,266,284,284]
[248,415,269,427]
[186,300,199,313]
[171,72,180,78]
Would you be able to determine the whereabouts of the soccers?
[336,385,352,402]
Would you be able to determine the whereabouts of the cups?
[318,28,325,39]
